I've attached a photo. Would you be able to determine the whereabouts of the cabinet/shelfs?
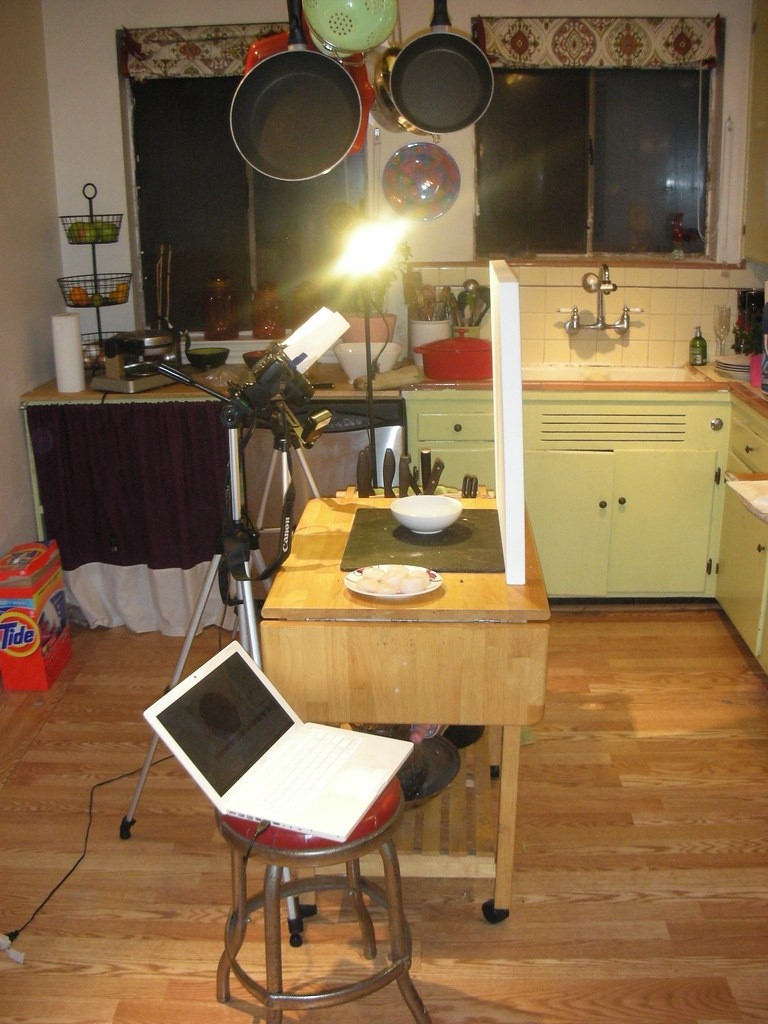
[57,183,134,375]
[405,391,768,676]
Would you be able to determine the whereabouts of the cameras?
[240,342,315,408]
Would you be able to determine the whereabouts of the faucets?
[597,263,617,329]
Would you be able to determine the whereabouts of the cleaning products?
[0,537,72,692]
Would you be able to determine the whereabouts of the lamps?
[329,215,411,488]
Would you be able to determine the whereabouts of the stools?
[214,774,433,1024]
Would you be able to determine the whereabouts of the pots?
[374,0,496,143]
[229,0,362,182]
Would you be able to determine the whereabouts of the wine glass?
[716,307,730,356]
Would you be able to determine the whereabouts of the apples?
[69,220,118,243]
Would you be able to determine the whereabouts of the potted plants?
[315,201,413,344]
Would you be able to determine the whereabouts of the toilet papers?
[51,313,86,393]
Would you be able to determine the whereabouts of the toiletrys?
[689,327,708,366]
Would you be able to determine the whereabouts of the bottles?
[689,326,707,366]
[292,282,320,331]
[251,289,286,340]
[205,279,238,339]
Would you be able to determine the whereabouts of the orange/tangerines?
[70,283,129,306]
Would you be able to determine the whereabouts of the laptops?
[144,641,415,843]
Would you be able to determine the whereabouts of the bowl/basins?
[390,494,463,534]
[412,337,493,380]
[334,342,400,385]
[243,351,267,367]
[185,348,229,368]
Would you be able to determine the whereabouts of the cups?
[750,353,762,388]
[409,318,452,366]
[451,325,481,339]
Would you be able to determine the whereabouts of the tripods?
[117,363,322,840]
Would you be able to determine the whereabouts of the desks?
[258,490,553,925]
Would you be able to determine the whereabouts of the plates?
[344,564,443,598]
[716,354,751,379]
[384,142,461,221]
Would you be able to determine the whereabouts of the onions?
[81,343,107,367]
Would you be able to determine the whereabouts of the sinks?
[522,364,706,382]
